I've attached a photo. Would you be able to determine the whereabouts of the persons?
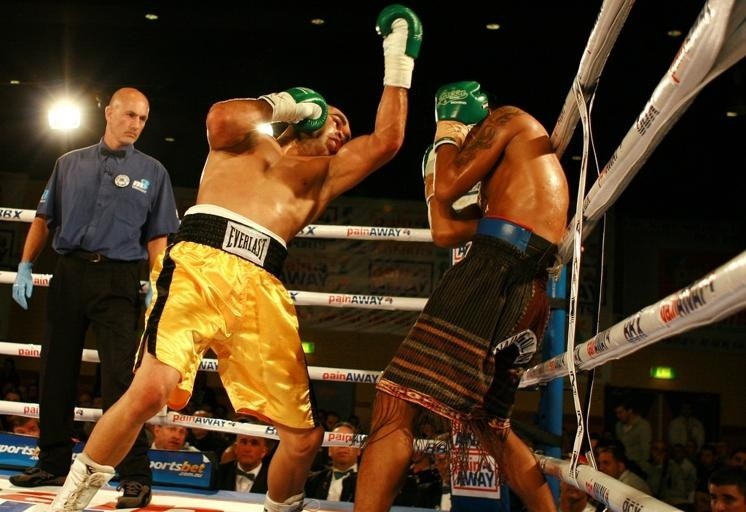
[354,82,569,512]
[12,88,182,509]
[559,401,746,512]
[2,359,452,512]
[45,4,423,512]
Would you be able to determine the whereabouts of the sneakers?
[51,453,115,512]
[116,479,152,508]
[263,489,320,512]
[10,466,65,486]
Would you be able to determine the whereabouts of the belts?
[69,250,142,265]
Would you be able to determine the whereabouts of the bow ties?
[334,469,353,479]
[235,468,254,480]
[101,147,126,157]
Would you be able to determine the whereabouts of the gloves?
[433,81,489,151]
[258,87,328,133]
[145,281,152,308]
[375,4,423,89]
[422,144,437,201]
[12,263,33,310]
